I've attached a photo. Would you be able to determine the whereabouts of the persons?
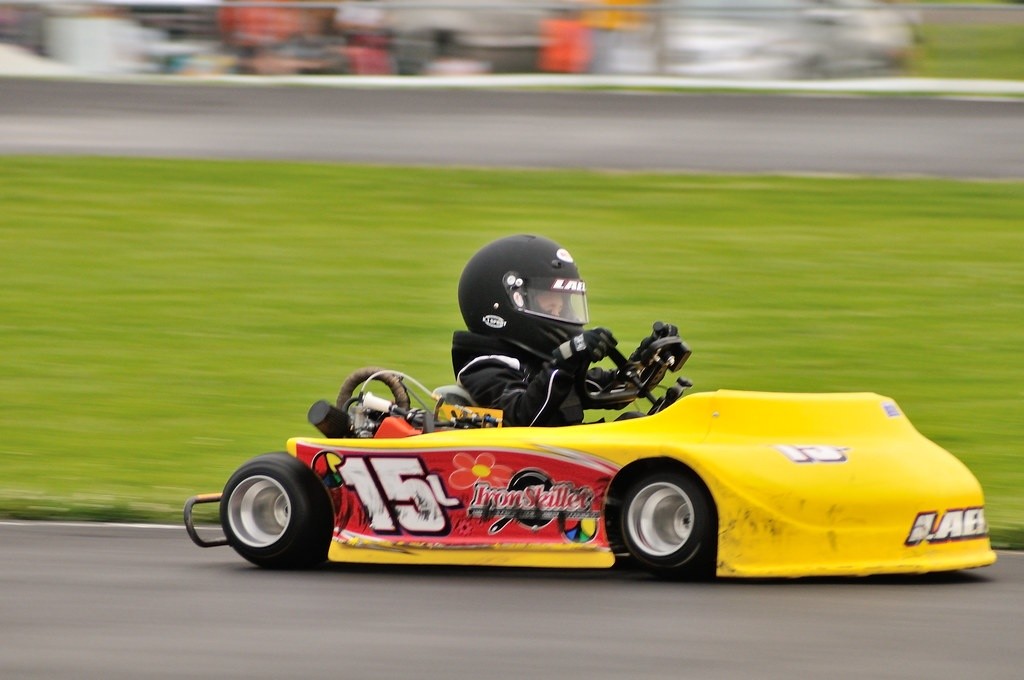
[0,0,934,81]
[449,232,678,428]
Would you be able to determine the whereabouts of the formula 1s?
[182,321,998,581]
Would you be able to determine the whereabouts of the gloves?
[627,319,679,367]
[553,326,619,371]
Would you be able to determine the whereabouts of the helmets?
[457,235,590,350]
